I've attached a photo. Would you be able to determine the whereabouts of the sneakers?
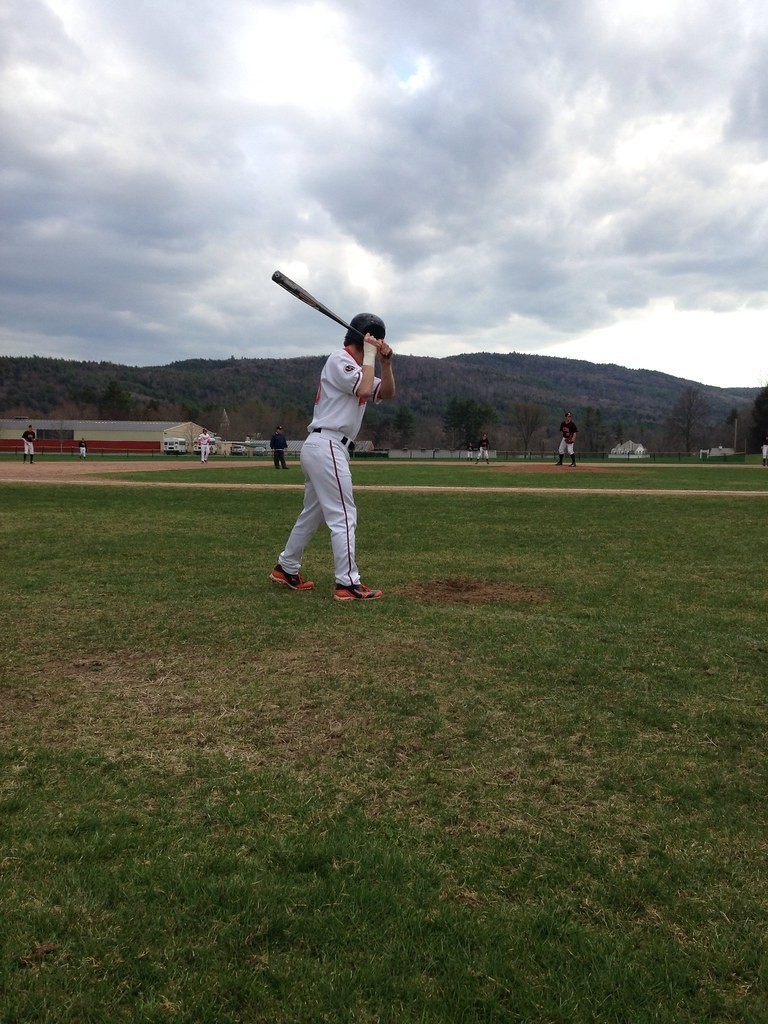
[283,467,288,469]
[333,583,383,601]
[475,461,479,464]
[569,463,576,467]
[555,462,562,465]
[487,462,489,464]
[204,460,208,462]
[269,564,314,590]
[202,461,204,463]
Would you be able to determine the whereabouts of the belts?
[313,427,356,451]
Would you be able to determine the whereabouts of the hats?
[565,412,573,417]
[276,426,284,430]
[483,434,487,437]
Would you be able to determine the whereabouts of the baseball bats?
[272,269,392,359]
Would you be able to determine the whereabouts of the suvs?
[232,445,248,456]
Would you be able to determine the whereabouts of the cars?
[253,447,268,456]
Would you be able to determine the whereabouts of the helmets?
[348,313,386,342]
[203,428,207,433]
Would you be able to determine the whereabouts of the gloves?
[565,437,573,444]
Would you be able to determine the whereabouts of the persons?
[269,313,396,602]
[761,435,768,467]
[474,434,489,464]
[22,424,35,464]
[467,442,473,461]
[198,428,211,464]
[556,412,578,467]
[270,425,289,469]
[79,438,87,459]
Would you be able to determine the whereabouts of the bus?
[193,438,218,455]
[162,437,190,455]
